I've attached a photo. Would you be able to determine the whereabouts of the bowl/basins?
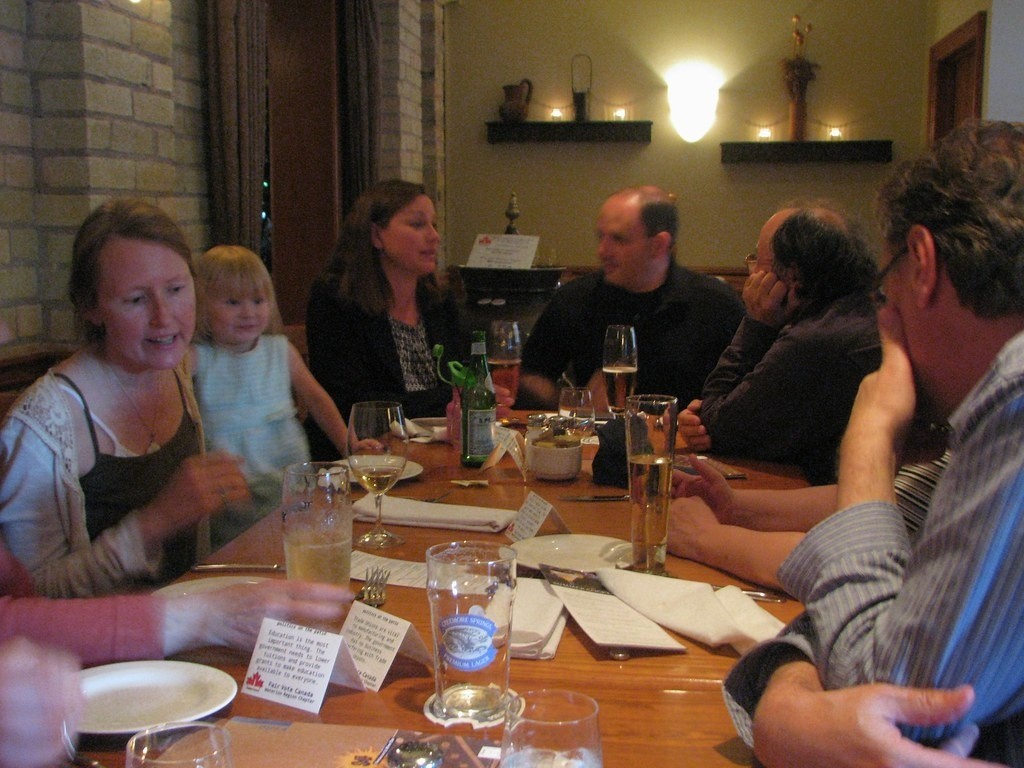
[527,444,585,480]
[447,263,568,290]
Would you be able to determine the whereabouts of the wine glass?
[488,320,521,423]
[602,325,639,419]
[347,402,410,548]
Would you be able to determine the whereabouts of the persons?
[183,245,385,557]
[636,415,951,604]
[0,576,355,768]
[675,194,947,487]
[305,179,514,464]
[0,197,251,596]
[509,185,745,417]
[721,118,1024,768]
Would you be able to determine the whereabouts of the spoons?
[387,742,444,768]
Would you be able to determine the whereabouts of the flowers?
[780,15,821,102]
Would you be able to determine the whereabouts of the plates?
[70,660,237,733]
[149,576,284,599]
[330,455,423,482]
[510,533,636,571]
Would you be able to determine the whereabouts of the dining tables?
[389,416,447,444]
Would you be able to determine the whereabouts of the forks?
[355,565,391,611]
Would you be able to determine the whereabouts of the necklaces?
[103,359,161,455]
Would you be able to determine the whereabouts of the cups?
[426,541,517,719]
[626,395,678,578]
[501,689,602,768]
[282,462,352,592]
[560,387,595,436]
[125,720,233,768]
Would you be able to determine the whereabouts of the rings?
[676,419,680,429]
[219,492,235,519]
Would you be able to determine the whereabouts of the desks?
[73,410,813,768]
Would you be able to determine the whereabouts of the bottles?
[524,412,568,479]
[460,330,495,466]
[446,388,463,451]
[593,420,658,486]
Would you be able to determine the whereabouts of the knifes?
[558,492,630,501]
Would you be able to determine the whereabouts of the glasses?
[745,254,772,270]
[867,245,910,309]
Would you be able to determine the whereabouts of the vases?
[789,102,806,142]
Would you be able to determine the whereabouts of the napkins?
[351,492,518,533]
[482,577,568,661]
[596,568,787,656]
